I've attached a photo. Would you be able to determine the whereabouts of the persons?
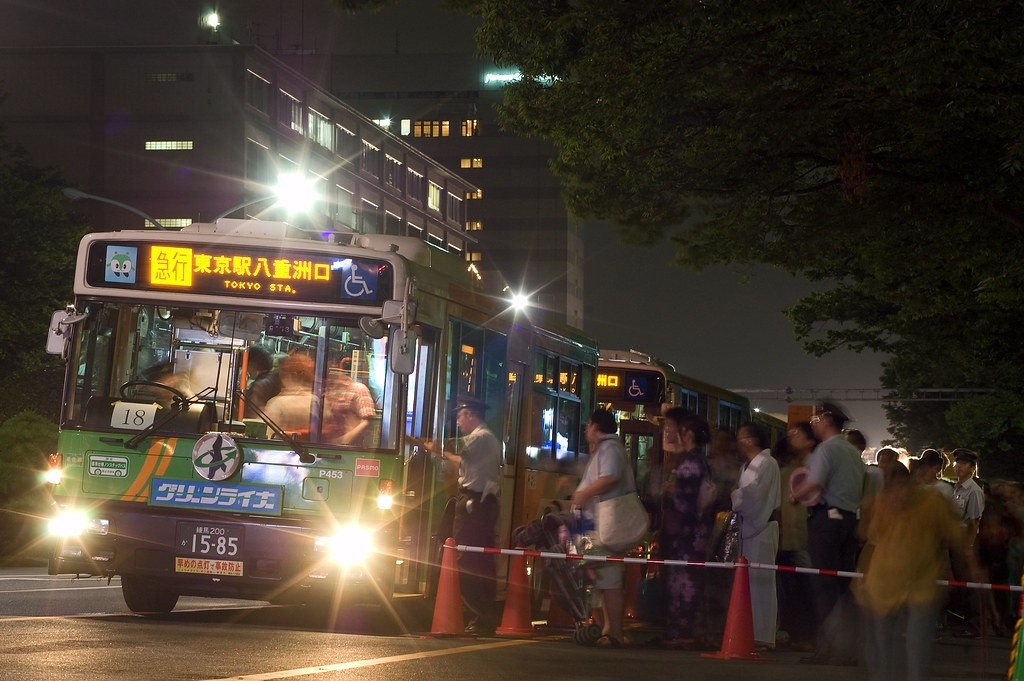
[137,363,198,400]
[233,346,374,447]
[571,406,1024,680]
[405,397,503,632]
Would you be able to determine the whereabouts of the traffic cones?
[698,555,775,663]
[494,544,540,639]
[418,538,479,641]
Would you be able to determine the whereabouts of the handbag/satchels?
[597,492,650,552]
[636,573,673,610]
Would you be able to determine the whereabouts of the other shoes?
[592,635,624,648]
[464,618,487,636]
[664,637,694,646]
[755,641,776,652]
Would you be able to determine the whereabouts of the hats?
[917,449,943,467]
[953,448,978,463]
[453,396,490,411]
[816,402,848,422]
[143,363,172,382]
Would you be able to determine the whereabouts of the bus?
[41,454,120,582]
[596,348,750,493]
[751,410,789,458]
[47,217,600,626]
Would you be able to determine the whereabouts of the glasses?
[735,435,753,441]
